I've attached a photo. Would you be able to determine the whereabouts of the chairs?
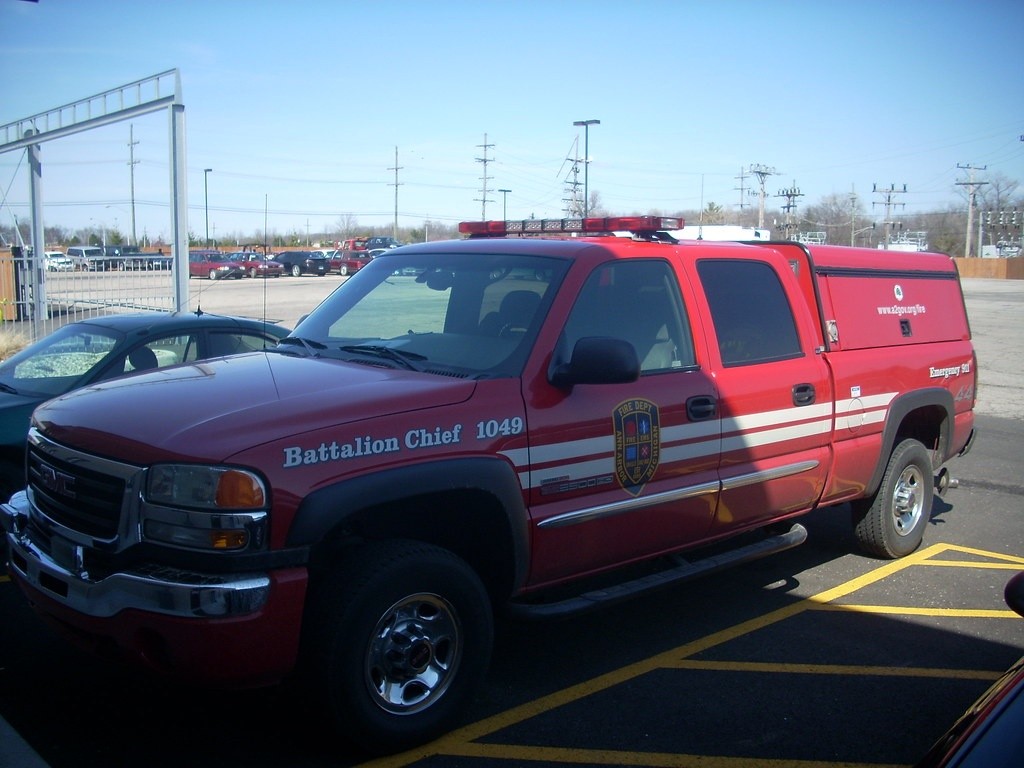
[129,347,158,370]
[478,281,682,373]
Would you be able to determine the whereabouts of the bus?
[343,237,368,250]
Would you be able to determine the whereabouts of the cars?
[66,245,171,273]
[0,305,386,494]
[189,251,246,280]
[227,252,285,280]
[369,249,391,259]
[45,250,76,272]
[365,237,403,249]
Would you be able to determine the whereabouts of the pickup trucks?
[271,250,333,278]
[326,249,375,276]
[0,218,979,747]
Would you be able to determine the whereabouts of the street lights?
[204,167,213,250]
[572,117,602,217]
[498,187,512,220]
